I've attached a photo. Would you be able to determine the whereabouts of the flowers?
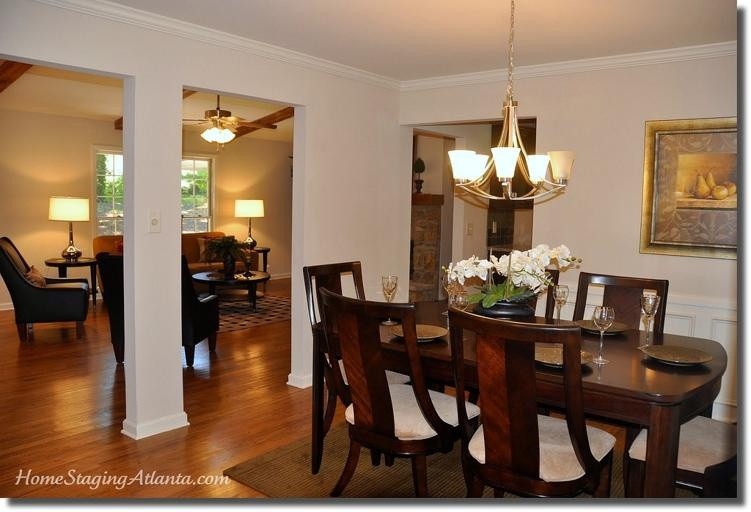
[441,244,581,308]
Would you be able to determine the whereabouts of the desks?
[46,257,98,313]
[255,247,270,284]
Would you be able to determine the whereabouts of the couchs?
[93,232,258,299]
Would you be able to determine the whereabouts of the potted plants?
[204,236,250,280]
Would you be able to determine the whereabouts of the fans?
[182,95,277,130]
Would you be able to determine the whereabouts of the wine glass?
[381,275,398,326]
[590,307,616,365]
[553,285,569,325]
[440,273,470,342]
[637,294,661,350]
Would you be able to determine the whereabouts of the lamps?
[49,195,90,260]
[447,0,576,201]
[201,122,237,144]
[234,200,264,250]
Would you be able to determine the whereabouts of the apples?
[724,182,737,195]
[712,185,728,199]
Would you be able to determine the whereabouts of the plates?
[532,319,713,369]
[390,323,449,343]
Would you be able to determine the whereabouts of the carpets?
[216,293,291,334]
[223,422,698,498]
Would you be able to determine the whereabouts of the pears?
[706,171,715,194]
[695,174,709,199]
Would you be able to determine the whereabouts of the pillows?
[197,236,234,263]
[25,265,46,288]
[113,240,123,254]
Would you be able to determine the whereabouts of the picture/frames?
[640,117,737,261]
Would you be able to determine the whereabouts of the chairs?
[303,261,737,498]
[96,252,219,367]
[0,237,89,343]
